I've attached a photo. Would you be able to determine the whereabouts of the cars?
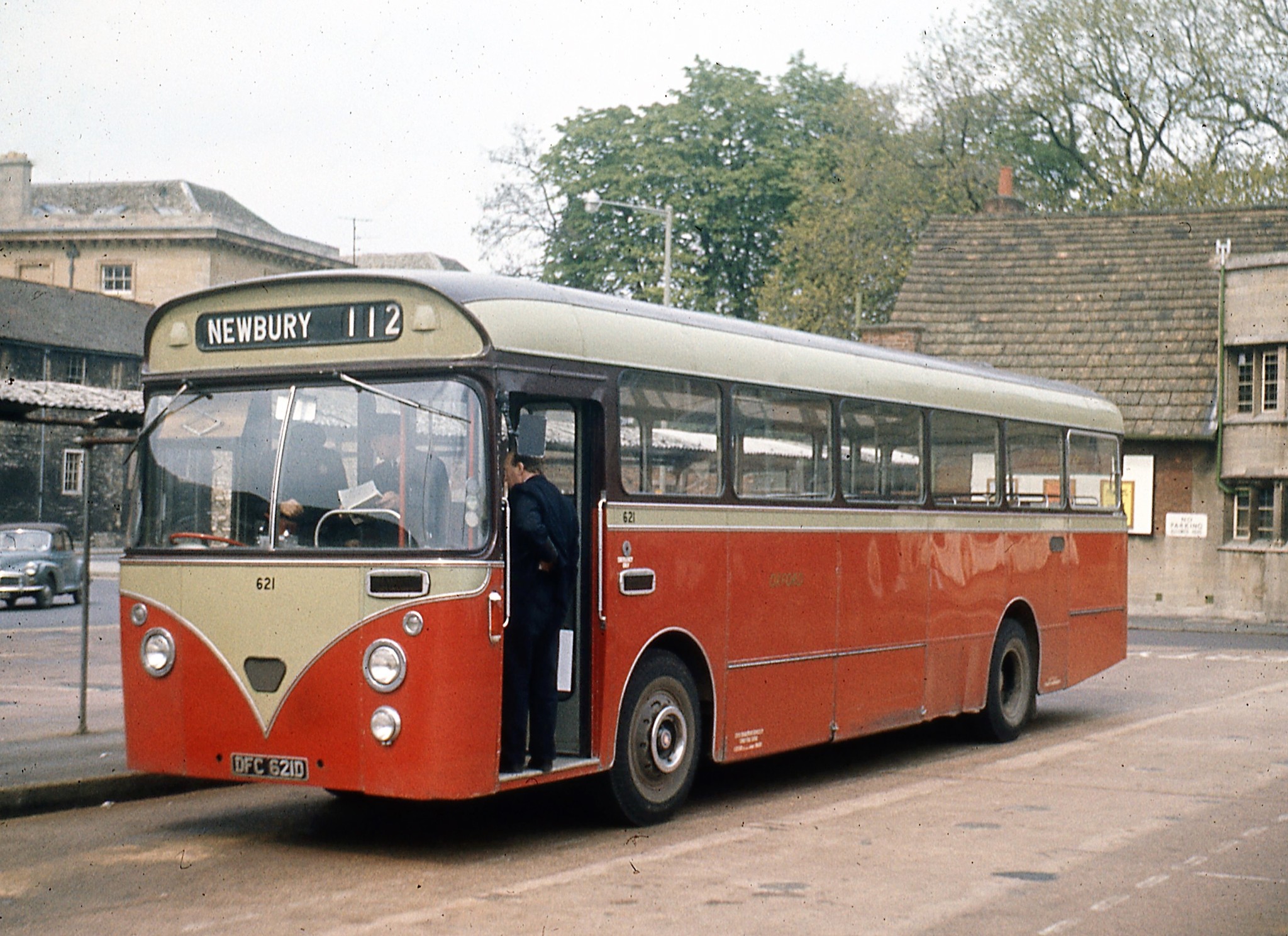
[1,521,86,609]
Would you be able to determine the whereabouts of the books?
[348,492,385,523]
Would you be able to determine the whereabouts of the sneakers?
[499,756,524,773]
[528,756,553,772]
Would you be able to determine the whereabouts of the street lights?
[583,193,673,308]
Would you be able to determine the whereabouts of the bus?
[114,266,1128,825]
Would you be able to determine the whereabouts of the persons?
[485,443,581,773]
[253,422,351,551]
[335,408,454,553]
